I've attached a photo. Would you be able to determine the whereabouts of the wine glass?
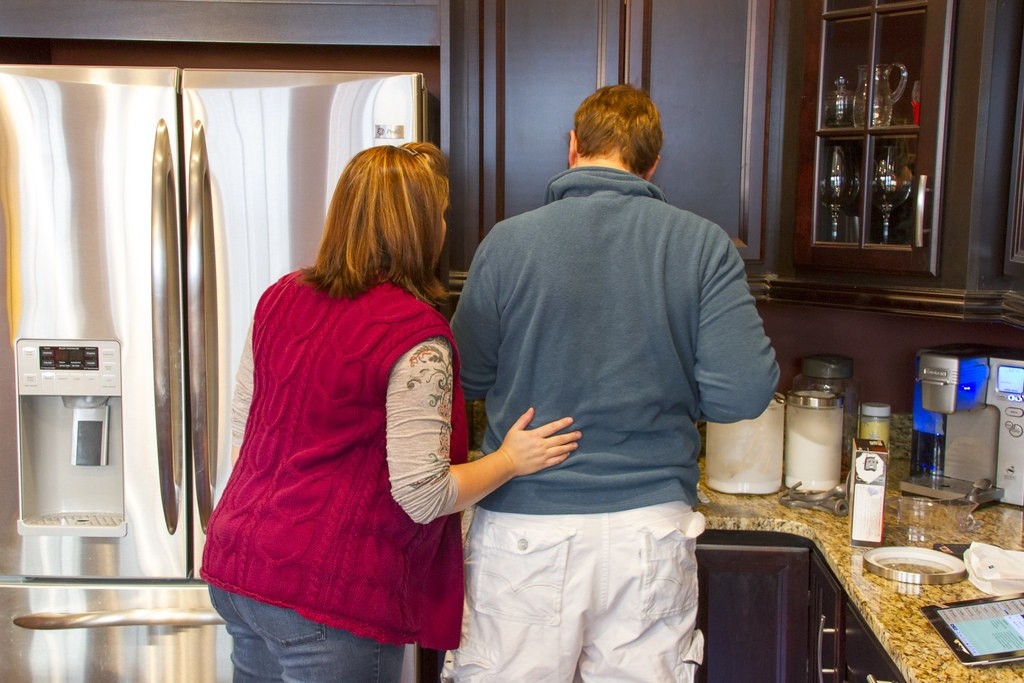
[818,144,862,242]
[867,146,915,246]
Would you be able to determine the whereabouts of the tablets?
[920,592,1024,665]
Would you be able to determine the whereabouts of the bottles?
[792,355,858,482]
[860,401,891,453]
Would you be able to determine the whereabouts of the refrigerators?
[1,67,424,683]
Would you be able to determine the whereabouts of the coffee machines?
[897,344,1024,510]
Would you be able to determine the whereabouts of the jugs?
[852,61,907,129]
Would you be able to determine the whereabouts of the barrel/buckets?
[704,393,786,495]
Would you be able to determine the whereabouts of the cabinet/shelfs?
[437,529,907,682]
[448,0,1024,332]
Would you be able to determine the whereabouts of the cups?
[822,74,854,127]
[783,392,844,492]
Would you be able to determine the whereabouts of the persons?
[449,83,781,683]
[198,142,582,683]
